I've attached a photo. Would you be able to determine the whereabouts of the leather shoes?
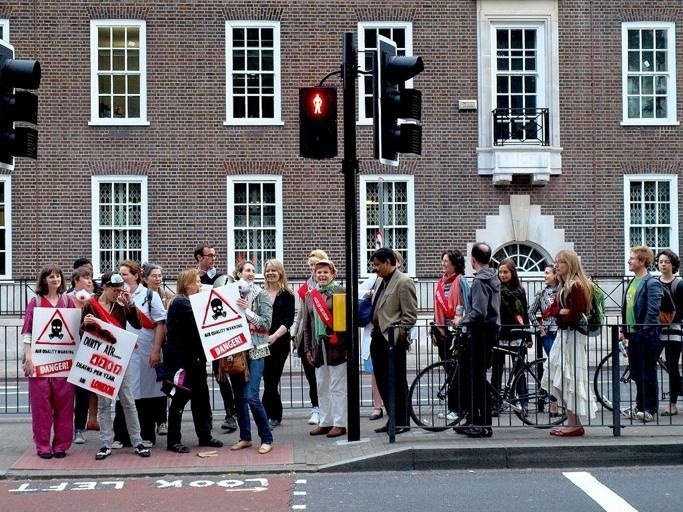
[308,407,409,437]
[554,427,584,436]
[491,405,498,417]
[453,421,470,433]
[522,408,528,417]
[462,426,492,437]
[37,415,278,460]
[550,405,558,417]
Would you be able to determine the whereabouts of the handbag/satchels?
[216,352,250,382]
[358,295,372,327]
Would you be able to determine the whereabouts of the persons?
[23,242,682,464]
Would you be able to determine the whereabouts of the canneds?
[456,305,463,316]
[619,341,628,358]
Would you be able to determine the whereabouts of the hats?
[74,259,89,269]
[315,259,337,279]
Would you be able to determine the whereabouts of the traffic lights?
[372,35,425,168]
[296,85,338,162]
[0,39,41,170]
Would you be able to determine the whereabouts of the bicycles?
[593,322,681,422]
[402,314,571,434]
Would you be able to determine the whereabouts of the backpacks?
[644,277,676,324]
[558,280,604,337]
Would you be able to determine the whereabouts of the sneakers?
[636,410,656,421]
[446,411,458,420]
[437,410,450,418]
[661,408,677,416]
[620,407,638,418]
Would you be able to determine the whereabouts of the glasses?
[203,254,216,258]
